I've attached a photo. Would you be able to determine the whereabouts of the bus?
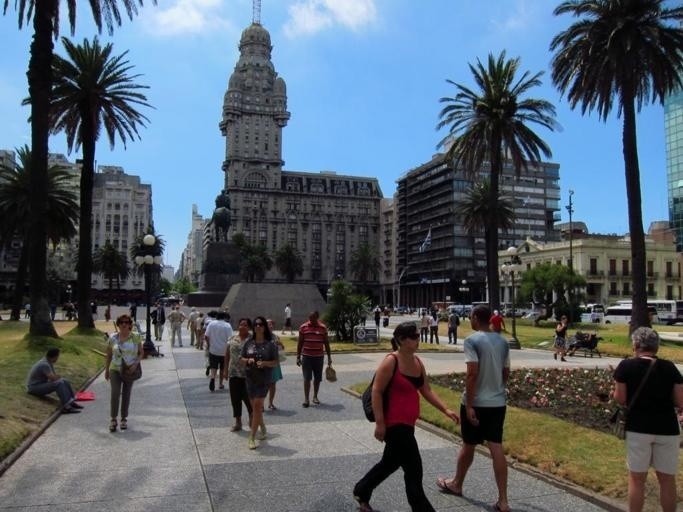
[189,292,224,307]
[159,298,179,306]
[615,300,677,325]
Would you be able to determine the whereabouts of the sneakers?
[313,396,319,403]
[302,401,309,407]
[64,408,80,413]
[206,365,276,449]
[72,402,83,408]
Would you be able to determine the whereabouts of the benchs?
[566,338,603,359]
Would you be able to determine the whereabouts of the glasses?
[119,320,130,324]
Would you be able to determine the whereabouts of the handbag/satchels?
[362,387,388,422]
[607,404,626,439]
[456,316,459,326]
[121,359,142,382]
[326,367,337,381]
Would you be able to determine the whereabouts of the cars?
[394,302,540,319]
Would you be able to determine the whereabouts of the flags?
[419,226,432,254]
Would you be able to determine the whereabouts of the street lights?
[566,188,575,271]
[501,246,521,349]
[66,283,72,303]
[458,278,470,320]
[133,233,164,359]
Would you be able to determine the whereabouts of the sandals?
[120,419,127,429]
[109,420,117,431]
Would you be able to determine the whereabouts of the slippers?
[436,478,462,495]
[354,494,372,512]
[492,501,510,512]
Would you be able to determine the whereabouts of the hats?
[493,310,499,316]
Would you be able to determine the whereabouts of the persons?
[215,188,231,212]
[566,331,596,355]
[435,305,514,512]
[149,300,287,450]
[104,314,145,432]
[352,321,460,511]
[282,303,296,336]
[1,291,139,323]
[611,325,682,511]
[359,301,506,345]
[296,309,332,408]
[553,314,567,362]
[27,347,84,414]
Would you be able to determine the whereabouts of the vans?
[604,306,658,326]
[578,303,604,323]
